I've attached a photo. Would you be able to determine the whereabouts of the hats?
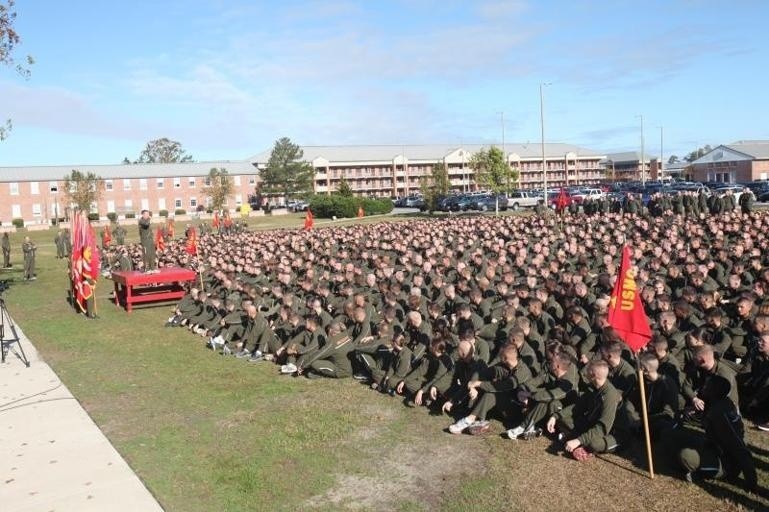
[288,198,312,212]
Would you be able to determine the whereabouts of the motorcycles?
[0,292,30,367]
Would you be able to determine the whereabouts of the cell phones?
[0,279,9,292]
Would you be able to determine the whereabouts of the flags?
[223,214,231,226]
[155,225,164,252]
[606,243,653,354]
[102,224,112,248]
[68,209,101,313]
[305,208,313,228]
[212,212,219,227]
[185,225,197,256]
[555,188,567,211]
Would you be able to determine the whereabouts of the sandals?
[259,192,288,211]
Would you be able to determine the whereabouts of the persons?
[21,236,38,282]
[100,222,111,249]
[161,223,169,242]
[63,227,72,257]
[534,187,756,215]
[138,209,160,274]
[1,232,13,269]
[217,211,249,235]
[56,229,66,260]
[198,220,212,235]
[108,213,768,484]
[112,222,129,245]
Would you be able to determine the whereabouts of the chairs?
[572,447,594,460]
[222,344,298,374]
[506,426,523,439]
[448,417,472,434]
[558,432,567,442]
[468,421,496,436]
[523,427,543,440]
[168,317,225,351]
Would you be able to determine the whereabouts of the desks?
[634,113,646,187]
[495,110,504,160]
[539,81,556,207]
